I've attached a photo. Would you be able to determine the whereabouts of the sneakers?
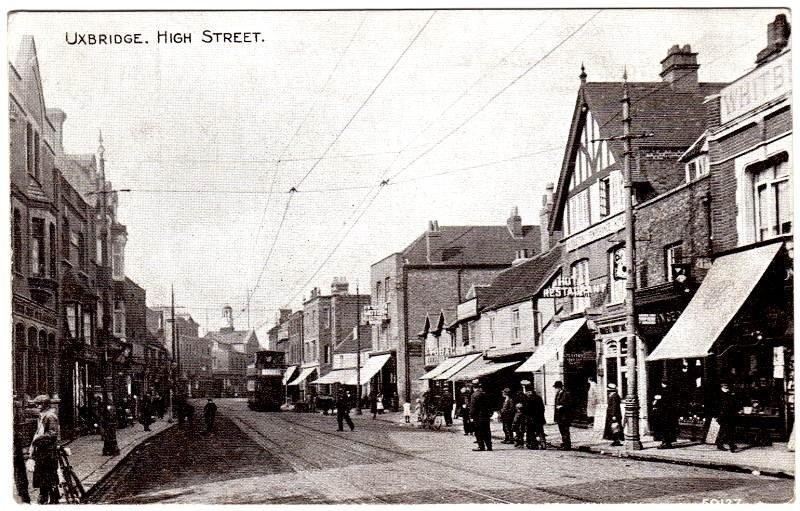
[717,442,743,454]
[465,433,492,452]
[656,442,673,450]
[610,440,623,447]
[335,427,356,432]
[500,439,572,451]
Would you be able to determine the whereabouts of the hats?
[605,383,617,391]
[469,379,483,388]
[502,388,512,396]
[553,382,565,387]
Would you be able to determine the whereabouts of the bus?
[243,350,286,411]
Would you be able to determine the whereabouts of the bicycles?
[418,404,446,432]
[32,432,88,504]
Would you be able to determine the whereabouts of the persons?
[13,397,29,504]
[29,393,60,504]
[401,376,548,453]
[83,392,220,459]
[333,386,357,433]
[553,380,574,453]
[651,376,679,452]
[376,392,385,416]
[712,379,738,455]
[601,380,626,447]
[369,387,382,419]
